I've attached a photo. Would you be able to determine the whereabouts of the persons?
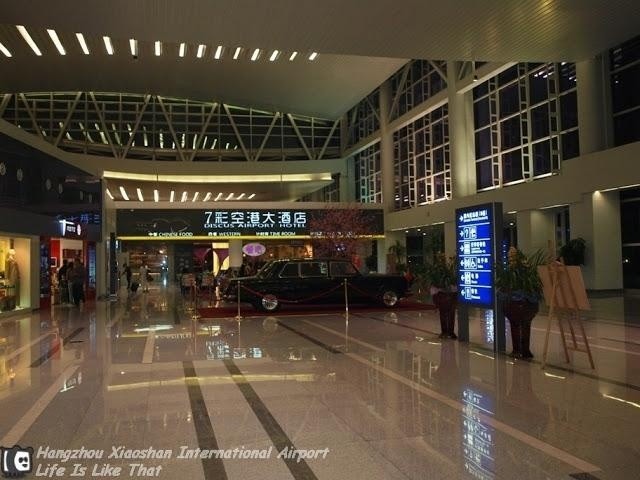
[503,246,540,360]
[120,263,132,289]
[74,259,81,270]
[144,265,152,293]
[58,259,68,286]
[5,248,25,312]
[431,266,460,340]
[139,266,146,293]
[65,262,81,305]
[222,261,266,280]
[58,273,69,305]
[76,263,88,305]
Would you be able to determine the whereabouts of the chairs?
[302,263,321,276]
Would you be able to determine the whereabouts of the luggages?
[131,282,139,292]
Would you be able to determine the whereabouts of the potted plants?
[493,244,560,358]
[406,248,459,340]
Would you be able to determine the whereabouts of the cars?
[224,257,409,314]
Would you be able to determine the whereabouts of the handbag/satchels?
[146,275,154,282]
